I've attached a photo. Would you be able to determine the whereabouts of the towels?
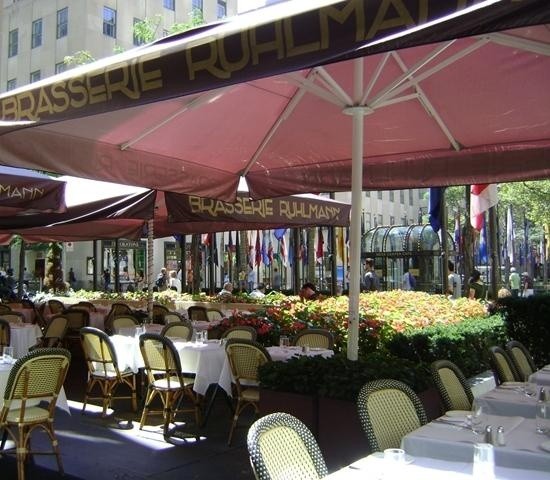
[414,413,525,444]
[483,389,529,402]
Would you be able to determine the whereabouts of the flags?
[174,228,350,267]
[475,212,488,266]
[451,216,463,261]
[503,208,550,267]
[427,186,443,233]
[470,183,499,232]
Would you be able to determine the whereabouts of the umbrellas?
[0,174,352,322]
[0,0,550,363]
[1,164,67,224]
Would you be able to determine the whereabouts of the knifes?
[432,419,472,431]
[497,386,520,391]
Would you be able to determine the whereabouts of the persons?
[522,271,533,297]
[249,284,267,299]
[509,266,521,290]
[169,271,182,293]
[69,267,76,284]
[157,267,168,293]
[219,282,233,296]
[345,266,350,289]
[364,258,380,294]
[272,268,281,293]
[468,269,487,299]
[23,267,30,288]
[447,261,461,298]
[224,272,229,283]
[301,282,318,301]
[402,268,416,291]
[105,269,111,289]
[247,265,255,292]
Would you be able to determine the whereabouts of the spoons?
[503,382,528,387]
[441,417,482,425]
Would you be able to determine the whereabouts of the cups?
[278,335,287,349]
[473,399,489,435]
[204,330,209,343]
[195,331,202,345]
[134,325,140,334]
[281,338,289,353]
[136,328,142,338]
[384,450,405,479]
[3,347,12,364]
[537,402,550,434]
[524,367,540,398]
[474,443,495,479]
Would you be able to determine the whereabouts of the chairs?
[69,302,98,312]
[220,326,257,345]
[188,305,207,322]
[505,340,537,382]
[161,312,183,323]
[487,345,521,387]
[138,332,204,437]
[32,306,46,329]
[205,307,224,322]
[78,325,139,420]
[29,314,70,351]
[289,328,334,351]
[111,302,133,315]
[103,304,121,331]
[246,411,329,480]
[158,321,193,343]
[222,337,277,449]
[355,377,428,454]
[147,304,169,323]
[111,316,139,334]
[38,300,65,319]
[0,318,11,355]
[0,311,26,325]
[20,299,35,309]
[62,308,89,362]
[0,346,73,480]
[430,359,475,412]
[0,304,11,314]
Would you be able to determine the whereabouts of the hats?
[521,271,530,277]
[509,266,517,272]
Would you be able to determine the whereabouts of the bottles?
[539,390,545,403]
[540,386,546,401]
[496,426,506,445]
[483,424,493,445]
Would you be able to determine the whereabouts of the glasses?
[363,263,366,266]
